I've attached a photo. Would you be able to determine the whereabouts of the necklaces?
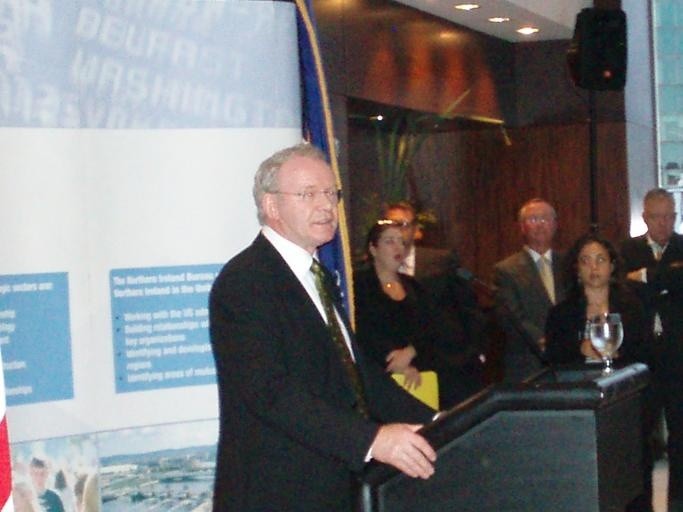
[379,281,397,287]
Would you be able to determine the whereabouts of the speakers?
[569,7,628,92]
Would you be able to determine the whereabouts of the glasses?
[377,220,405,228]
[524,215,554,224]
[278,190,343,205]
[577,257,606,265]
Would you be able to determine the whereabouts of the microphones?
[457,268,510,306]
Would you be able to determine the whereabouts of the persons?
[9,444,96,512]
[492,197,579,384]
[353,200,483,385]
[545,233,652,371]
[208,143,445,512]
[354,220,468,422]
[612,189,683,512]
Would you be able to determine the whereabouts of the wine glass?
[588,312,625,380]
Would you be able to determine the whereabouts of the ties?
[540,257,555,303]
[310,258,369,420]
[401,261,412,274]
